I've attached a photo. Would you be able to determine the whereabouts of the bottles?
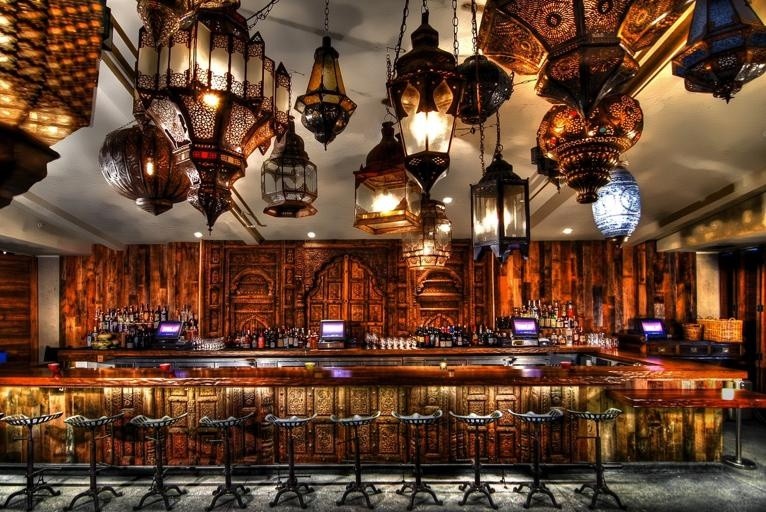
[497,314,512,329]
[544,329,621,350]
[512,299,579,328]
[85,303,166,350]
[176,303,197,323]
[472,323,501,348]
[234,327,319,352]
[417,323,470,348]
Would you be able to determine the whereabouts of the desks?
[610,388,766,470]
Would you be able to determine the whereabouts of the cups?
[364,330,417,349]
[304,362,314,369]
[440,362,447,368]
[159,362,171,374]
[48,361,60,372]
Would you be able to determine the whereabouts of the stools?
[507,407,565,509]
[328,411,382,509]
[266,414,319,509]
[389,411,444,508]
[197,412,256,510]
[568,407,627,511]
[61,413,122,512]
[448,411,502,509]
[1,414,62,512]
[130,414,190,511]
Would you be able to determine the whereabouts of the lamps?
[0,1,103,147]
[129,0,290,236]
[260,133,318,215]
[294,0,357,151]
[98,123,190,215]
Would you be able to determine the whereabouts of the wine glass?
[191,336,227,352]
